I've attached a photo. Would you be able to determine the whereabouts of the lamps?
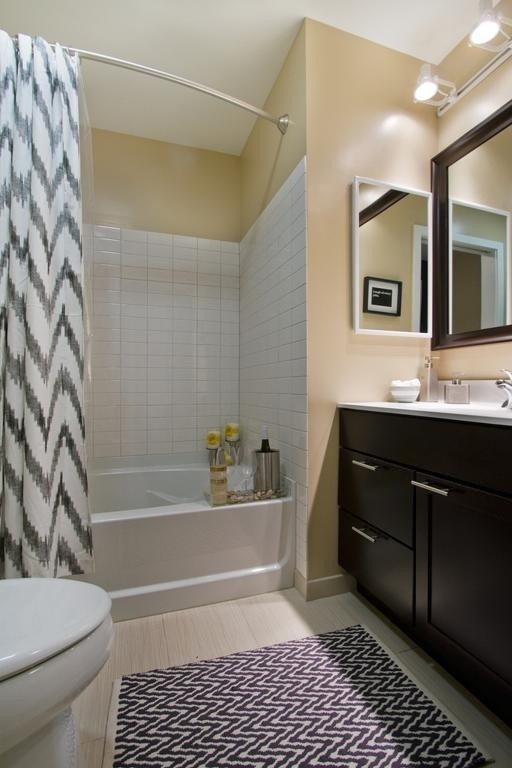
[411,0,512,120]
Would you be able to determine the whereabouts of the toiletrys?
[444,369,470,404]
[444,373,471,404]
[418,354,442,403]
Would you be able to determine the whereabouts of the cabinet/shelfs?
[334,408,510,737]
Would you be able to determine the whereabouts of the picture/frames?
[362,276,402,317]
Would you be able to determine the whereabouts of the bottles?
[260,424,271,451]
[210,464,227,506]
[444,380,472,405]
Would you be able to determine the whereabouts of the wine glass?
[216,446,238,493]
[236,446,257,491]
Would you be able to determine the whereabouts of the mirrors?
[349,173,432,337]
[358,191,427,331]
[429,99,512,351]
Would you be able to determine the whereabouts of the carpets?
[95,622,495,766]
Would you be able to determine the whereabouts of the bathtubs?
[63,463,298,625]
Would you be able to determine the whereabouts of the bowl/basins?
[389,385,421,403]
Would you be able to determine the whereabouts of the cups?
[225,440,241,465]
[206,447,218,466]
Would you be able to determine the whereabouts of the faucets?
[495,368,512,411]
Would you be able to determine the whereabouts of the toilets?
[0,574,116,768]
